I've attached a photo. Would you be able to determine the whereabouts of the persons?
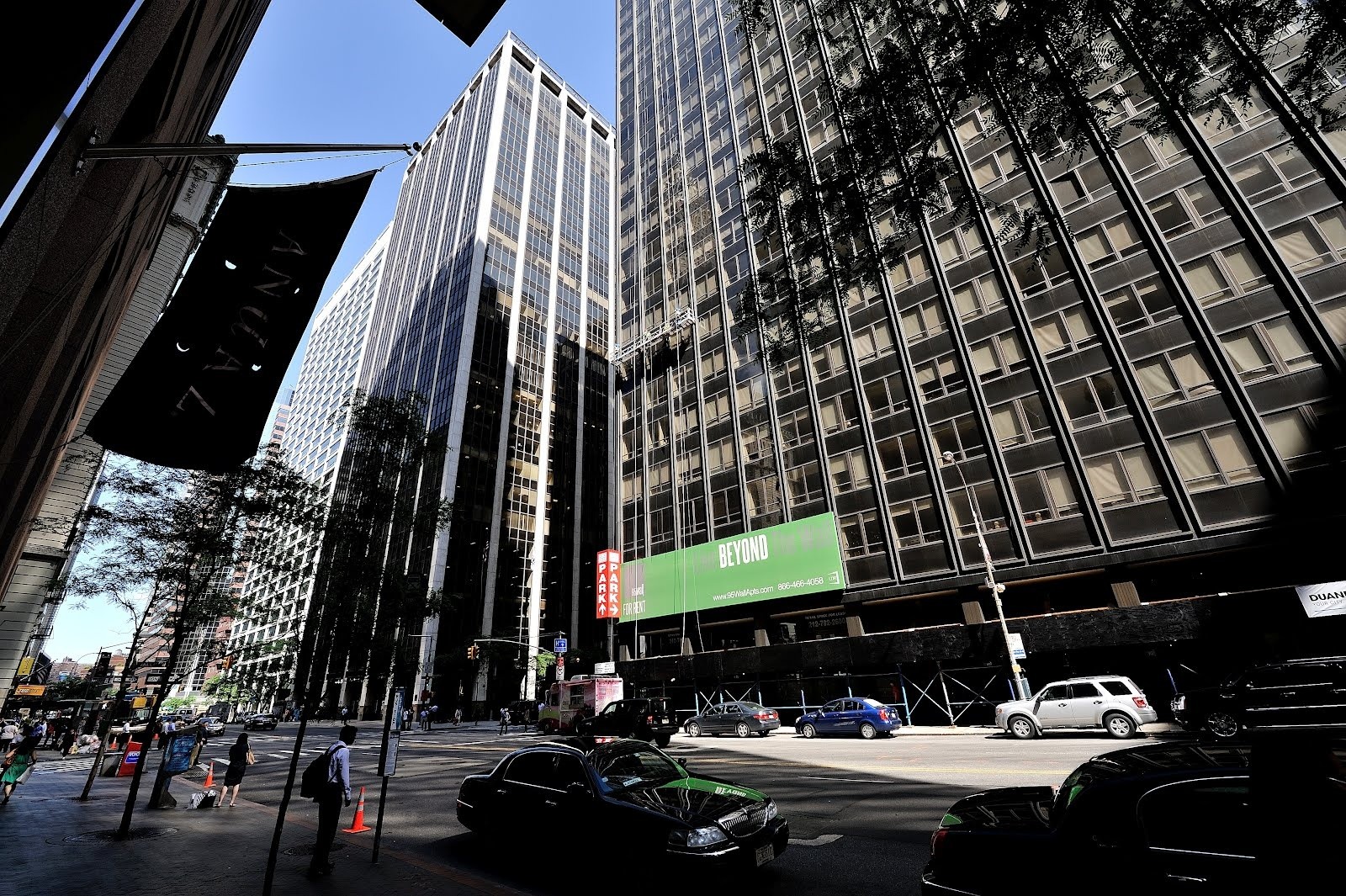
[158,718,209,769]
[572,710,585,736]
[217,733,251,807]
[117,719,132,752]
[497,700,545,735]
[0,716,85,808]
[401,702,484,732]
[285,705,348,725]
[310,726,358,874]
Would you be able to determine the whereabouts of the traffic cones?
[342,786,371,833]
[204,762,217,788]
[110,737,117,749]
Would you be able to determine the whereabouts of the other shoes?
[2,795,10,805]
[307,861,336,874]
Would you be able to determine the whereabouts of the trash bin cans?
[99,752,124,777]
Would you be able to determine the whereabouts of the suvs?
[1172,656,1346,737]
[996,675,1158,739]
[576,698,679,747]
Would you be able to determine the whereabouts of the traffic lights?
[468,646,480,660]
[221,655,240,669]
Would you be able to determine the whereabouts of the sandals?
[216,801,222,807]
[230,802,237,807]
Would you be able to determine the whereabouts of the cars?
[795,697,902,739]
[683,701,781,738]
[244,713,278,730]
[236,712,258,724]
[924,743,1346,896]
[194,716,226,736]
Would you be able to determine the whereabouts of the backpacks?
[299,744,347,798]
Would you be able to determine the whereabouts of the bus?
[55,695,157,744]
[456,736,789,896]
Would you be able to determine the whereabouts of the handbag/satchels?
[16,764,36,786]
[1,755,14,767]
[246,743,255,766]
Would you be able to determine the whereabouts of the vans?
[173,709,195,723]
